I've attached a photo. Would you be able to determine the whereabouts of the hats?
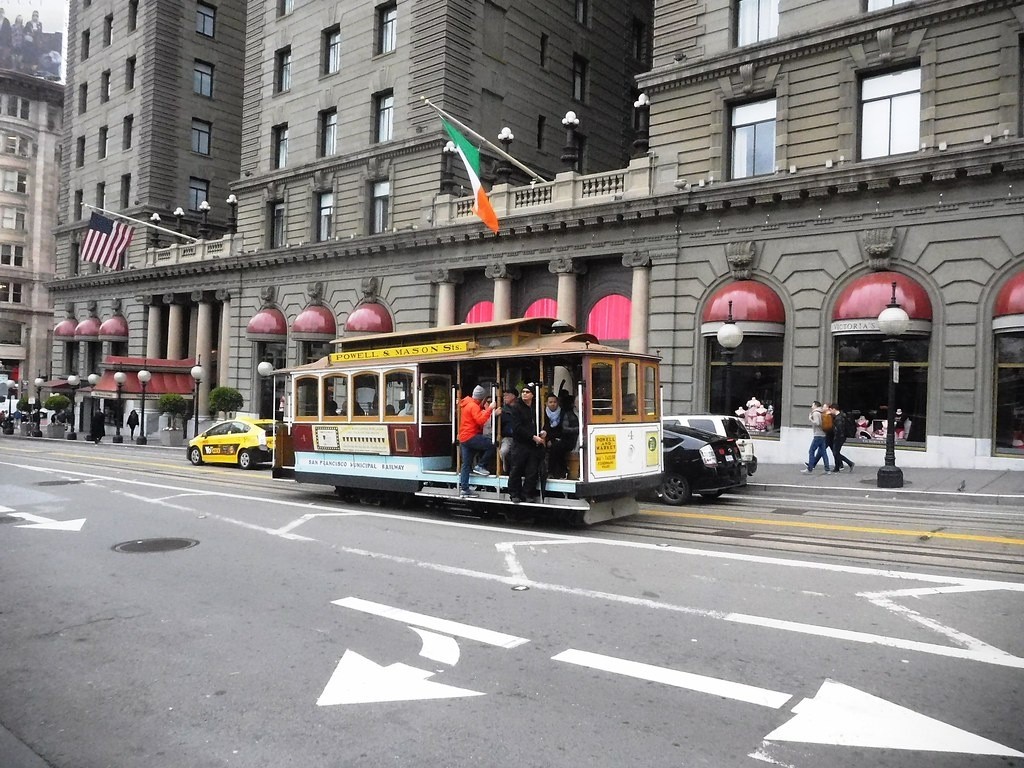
[503,388,519,398]
[472,385,487,401]
[521,386,535,397]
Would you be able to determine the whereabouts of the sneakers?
[473,465,490,476]
[460,489,479,498]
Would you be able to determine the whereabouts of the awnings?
[992,268,1024,333]
[53,318,128,342]
[830,270,934,336]
[699,280,785,339]
[245,301,393,341]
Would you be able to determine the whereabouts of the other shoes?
[850,462,854,472]
[801,468,812,474]
[831,470,840,474]
[839,467,844,470]
[823,468,831,475]
[523,496,534,503]
[804,461,815,470]
[512,496,520,502]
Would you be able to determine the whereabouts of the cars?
[186,418,280,471]
[6,413,28,424]
[641,423,748,507]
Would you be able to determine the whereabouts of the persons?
[894,408,908,439]
[0,411,5,427]
[0,8,44,73]
[51,412,61,425]
[91,411,105,444]
[14,410,21,427]
[481,385,636,503]
[328,394,413,416]
[127,410,139,440]
[458,386,496,498]
[735,397,774,432]
[800,400,855,474]
[855,416,868,438]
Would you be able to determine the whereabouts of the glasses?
[522,391,531,394]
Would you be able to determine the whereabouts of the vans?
[661,412,758,476]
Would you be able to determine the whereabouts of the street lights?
[190,353,206,438]
[6,379,16,434]
[86,373,100,441]
[112,361,127,443]
[876,280,916,489]
[135,359,152,444]
[32,377,44,438]
[66,373,80,441]
[717,299,743,414]
[257,348,274,420]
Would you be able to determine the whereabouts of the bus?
[271,317,665,525]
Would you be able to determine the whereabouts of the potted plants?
[16,392,41,436]
[44,395,70,439]
[156,392,188,447]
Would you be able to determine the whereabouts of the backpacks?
[814,409,832,430]
[839,413,857,438]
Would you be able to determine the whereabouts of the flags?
[439,116,498,234]
[80,213,135,271]
